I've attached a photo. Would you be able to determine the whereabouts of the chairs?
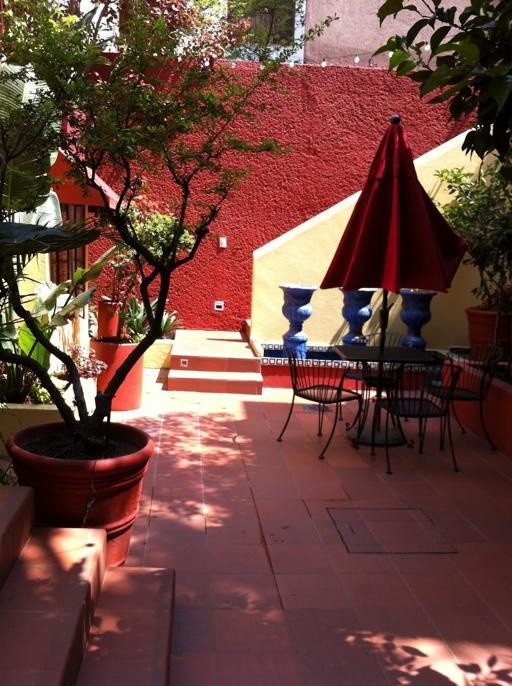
[277,333,503,473]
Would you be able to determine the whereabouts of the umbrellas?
[317,113,466,432]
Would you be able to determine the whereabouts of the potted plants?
[437,158,512,360]
[0,0,337,570]
